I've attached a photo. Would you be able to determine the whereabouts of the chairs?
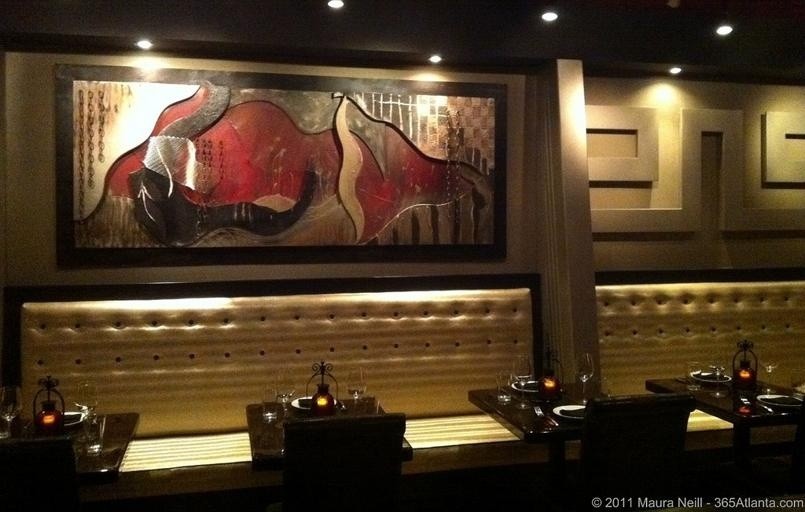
[583,391,697,510]
[0,436,78,512]
[284,413,406,511]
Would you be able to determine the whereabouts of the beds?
[645,377,805,510]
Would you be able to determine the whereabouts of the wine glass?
[276,367,298,411]
[78,381,98,418]
[0,384,24,444]
[709,350,728,398]
[759,341,781,394]
[348,366,368,407]
[512,352,533,409]
[575,351,594,404]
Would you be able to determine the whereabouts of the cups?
[688,361,706,390]
[84,416,106,457]
[494,372,513,405]
[262,399,279,424]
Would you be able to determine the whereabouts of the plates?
[553,404,586,421]
[689,371,733,383]
[290,395,337,412]
[510,380,539,393]
[59,411,89,426]
[756,393,804,407]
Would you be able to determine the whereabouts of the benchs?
[21,283,566,510]
[594,268,805,510]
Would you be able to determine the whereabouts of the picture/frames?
[54,63,509,268]
